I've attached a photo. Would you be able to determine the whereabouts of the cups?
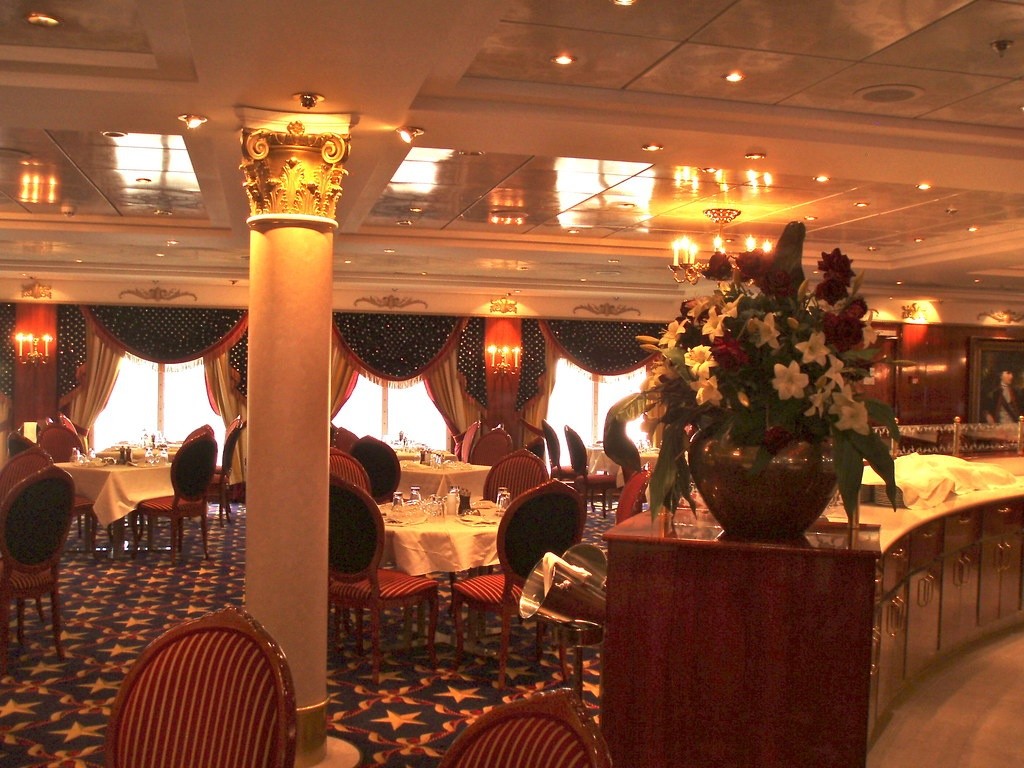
[497,487,511,509]
[406,493,448,517]
[74,449,80,461]
[393,492,403,511]
[449,485,460,501]
[410,487,422,501]
[447,494,456,515]
[88,447,95,459]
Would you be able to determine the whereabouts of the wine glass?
[146,446,154,463]
[158,447,169,461]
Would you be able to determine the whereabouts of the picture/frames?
[969,336,1024,425]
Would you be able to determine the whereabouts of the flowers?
[624,221,908,554]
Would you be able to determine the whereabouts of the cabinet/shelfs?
[599,498,1024,767]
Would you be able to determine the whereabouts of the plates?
[457,517,497,528]
[386,518,427,527]
[874,485,904,505]
[860,485,874,502]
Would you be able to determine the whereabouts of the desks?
[584,445,660,508]
[397,460,493,503]
[94,448,179,462]
[395,448,458,462]
[377,500,507,648]
[54,462,175,560]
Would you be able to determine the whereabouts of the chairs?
[0,412,656,768]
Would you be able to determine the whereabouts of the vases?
[689,411,838,541]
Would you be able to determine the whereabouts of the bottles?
[399,432,410,452]
[420,444,444,468]
[120,445,131,464]
[70,447,76,460]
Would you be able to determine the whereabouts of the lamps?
[16,332,49,368]
[397,126,425,143]
[489,344,520,378]
[668,208,771,288]
[293,93,324,109]
[177,113,208,129]
